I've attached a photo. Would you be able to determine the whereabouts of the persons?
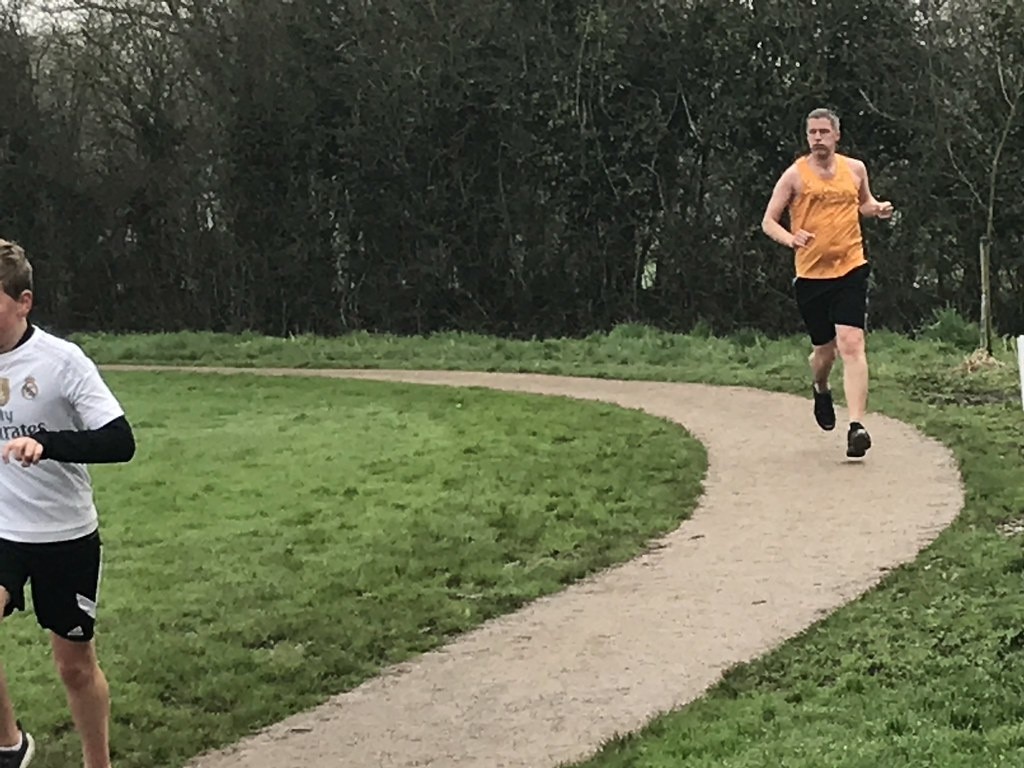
[0,239,137,768]
[762,109,894,460]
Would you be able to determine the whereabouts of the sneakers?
[812,381,835,431]
[847,429,871,457]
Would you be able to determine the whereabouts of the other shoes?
[0,719,35,768]
